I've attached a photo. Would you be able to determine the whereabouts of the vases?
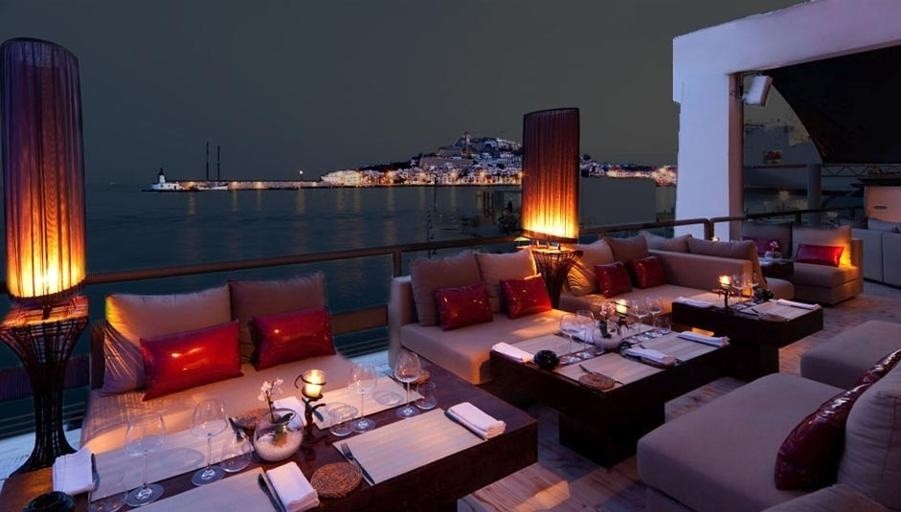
[592,320,623,349]
[253,408,304,462]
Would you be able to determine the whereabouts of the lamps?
[519,107,583,308]
[1,36,91,481]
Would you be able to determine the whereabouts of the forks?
[751,308,789,319]
[340,442,376,485]
[638,344,682,363]
[579,364,625,387]
[388,374,415,391]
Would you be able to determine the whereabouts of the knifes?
[301,397,324,422]
[89,454,100,493]
[257,473,282,512]
[444,412,485,441]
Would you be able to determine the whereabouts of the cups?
[414,381,439,409]
[655,315,672,334]
[324,402,359,436]
[221,429,253,472]
[592,334,605,355]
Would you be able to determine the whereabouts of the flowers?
[257,379,294,447]
[599,302,615,339]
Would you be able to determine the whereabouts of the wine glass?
[746,272,760,307]
[122,414,165,506]
[647,294,663,338]
[559,314,580,362]
[576,310,595,358]
[346,364,377,432]
[190,398,228,486]
[732,273,747,309]
[630,297,649,341]
[86,470,129,512]
[394,352,421,417]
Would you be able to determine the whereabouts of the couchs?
[760,241,864,306]
[636,318,901,511]
[387,250,753,399]
[79,322,379,453]
[851,228,901,286]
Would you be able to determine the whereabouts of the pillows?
[638,221,691,251]
[227,271,329,366]
[252,306,337,371]
[560,233,666,299]
[838,361,901,510]
[96,281,235,400]
[775,350,901,491]
[408,247,553,332]
[686,237,767,289]
[140,320,243,402]
[742,223,851,265]
[867,219,900,233]
